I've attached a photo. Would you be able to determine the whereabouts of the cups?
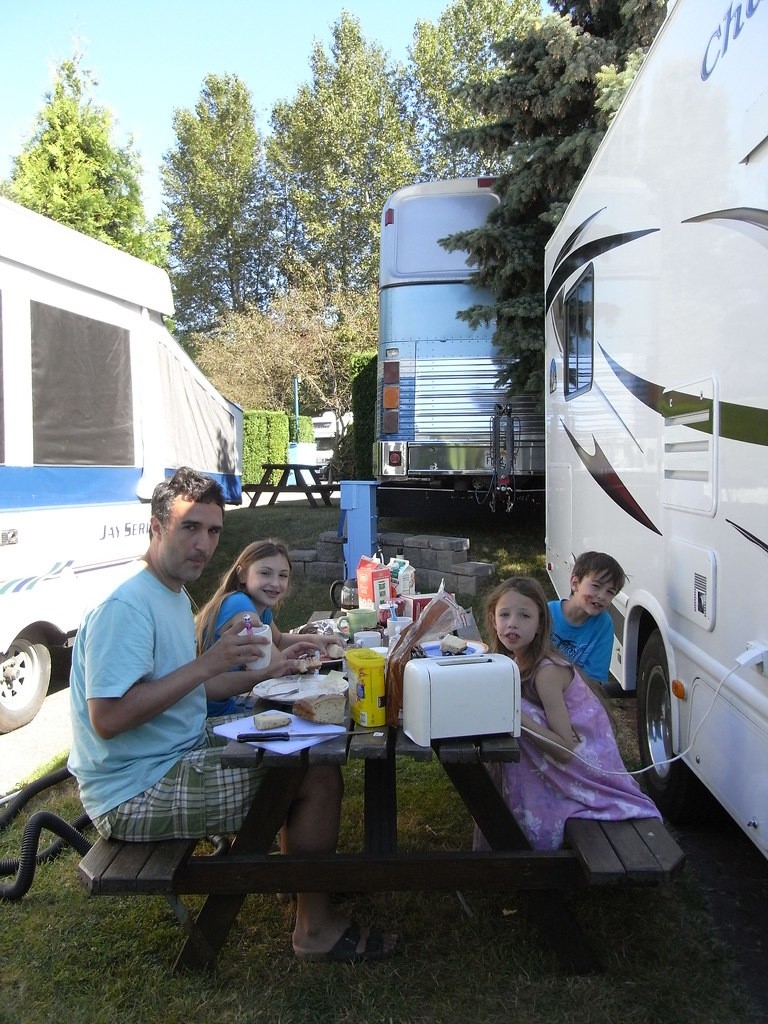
[387,618,413,644]
[380,603,398,627]
[237,625,273,671]
[355,631,380,650]
[338,609,377,639]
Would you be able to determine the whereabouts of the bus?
[369,172,549,506]
[542,1,768,870]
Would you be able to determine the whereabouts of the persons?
[65,463,404,963]
[193,542,351,899]
[548,548,630,687]
[470,574,664,855]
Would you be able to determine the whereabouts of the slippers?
[290,916,407,964]
[276,893,364,905]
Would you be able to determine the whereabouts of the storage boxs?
[400,593,456,621]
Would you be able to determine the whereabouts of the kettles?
[330,579,359,609]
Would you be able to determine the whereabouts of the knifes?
[236,730,375,742]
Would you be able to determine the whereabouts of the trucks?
[0,195,244,742]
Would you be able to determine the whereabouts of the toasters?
[403,653,522,746]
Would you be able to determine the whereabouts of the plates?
[252,675,349,702]
[297,649,347,663]
[417,640,489,658]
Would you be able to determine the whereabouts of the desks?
[170,611,616,977]
[248,464,334,509]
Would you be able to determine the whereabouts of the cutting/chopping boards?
[212,710,346,754]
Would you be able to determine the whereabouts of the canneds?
[379,604,398,628]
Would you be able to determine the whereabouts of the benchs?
[73,798,686,978]
[242,484,342,509]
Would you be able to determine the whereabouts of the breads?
[254,715,292,730]
[326,643,344,659]
[440,634,468,654]
[296,654,323,673]
[292,693,345,724]
